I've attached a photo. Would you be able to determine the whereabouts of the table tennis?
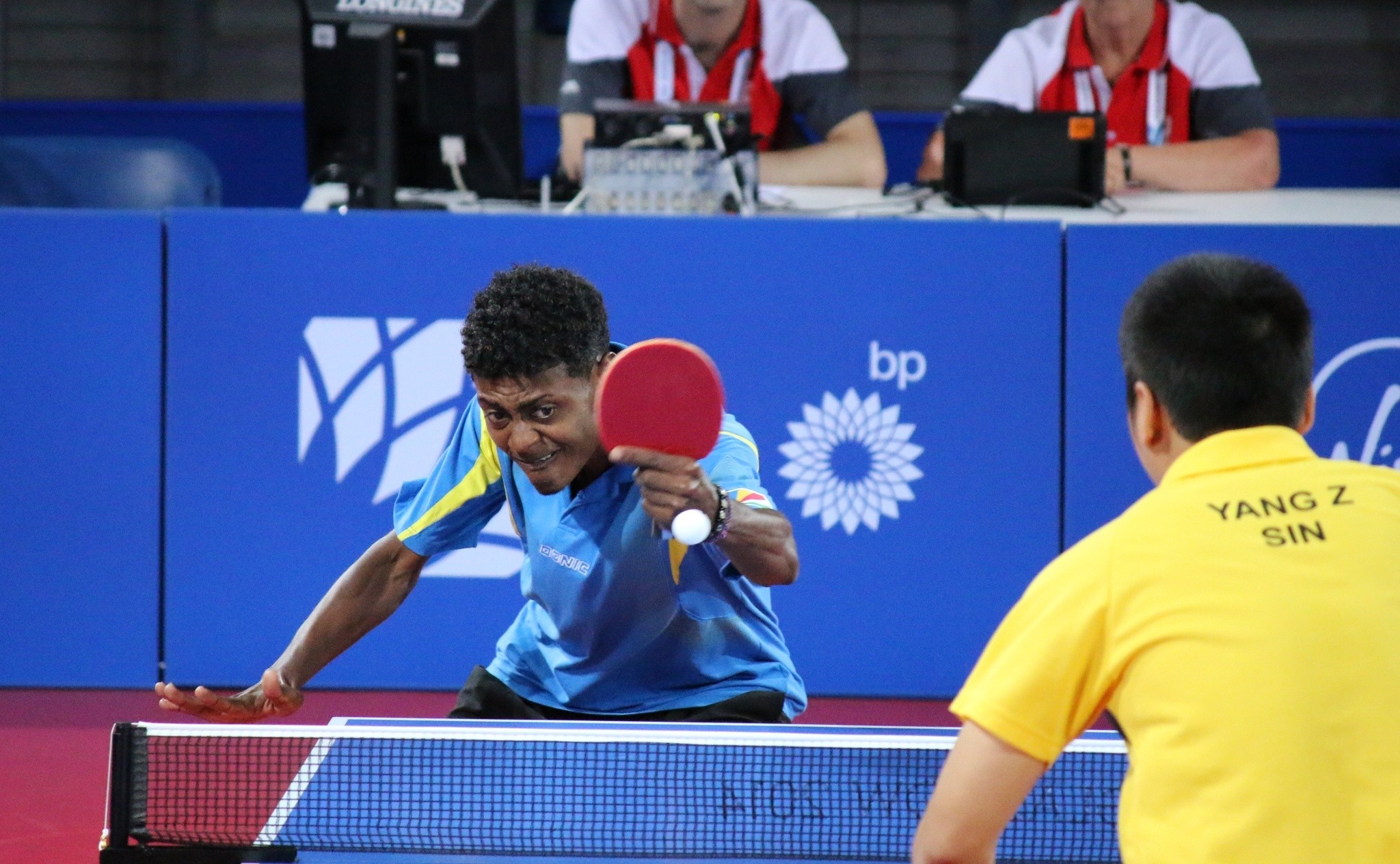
[671,509,711,546]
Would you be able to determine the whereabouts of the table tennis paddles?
[592,338,724,543]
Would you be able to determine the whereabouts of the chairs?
[0,133,222,226]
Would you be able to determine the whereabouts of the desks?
[90,715,1130,864]
[760,182,1400,227]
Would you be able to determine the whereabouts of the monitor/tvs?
[298,0,524,210]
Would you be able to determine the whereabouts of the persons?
[155,262,807,727]
[558,0,886,198]
[917,0,1283,193]
[899,250,1399,864]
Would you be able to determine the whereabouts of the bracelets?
[703,483,734,544]
[1119,145,1135,190]
